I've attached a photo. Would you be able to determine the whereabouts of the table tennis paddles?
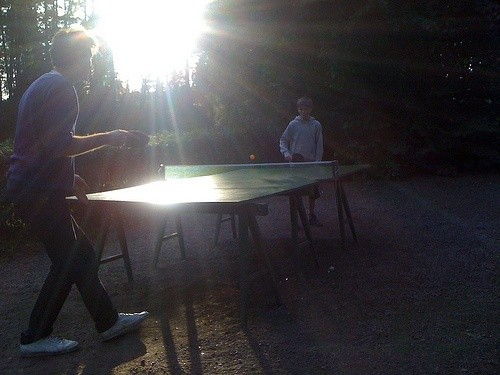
[292,153,304,162]
[126,130,149,148]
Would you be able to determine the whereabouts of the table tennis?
[250,155,255,160]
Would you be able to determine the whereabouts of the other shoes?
[99,311,148,341]
[19,336,78,354]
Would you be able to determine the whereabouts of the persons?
[279,96,324,229]
[4,27,150,358]
